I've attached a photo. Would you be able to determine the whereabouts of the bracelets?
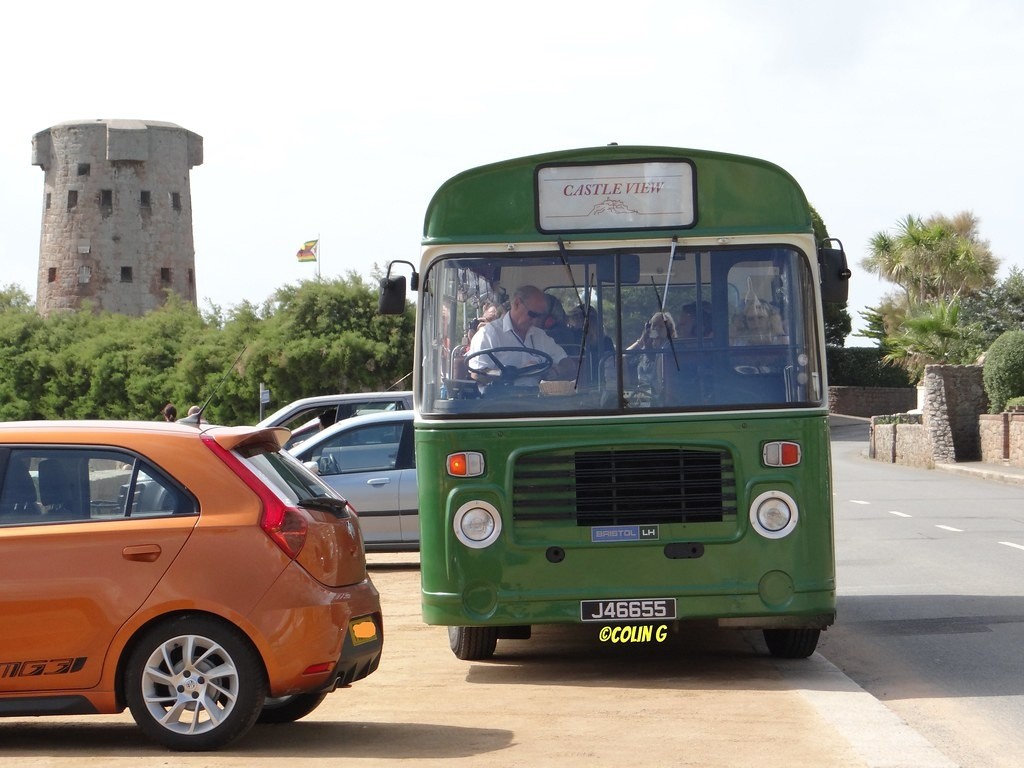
[637,340,643,348]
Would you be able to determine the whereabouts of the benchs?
[661,334,791,407]
[450,344,590,389]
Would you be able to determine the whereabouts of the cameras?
[468,319,484,331]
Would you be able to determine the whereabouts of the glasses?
[519,298,543,319]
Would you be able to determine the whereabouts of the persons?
[465,275,789,394]
[160,404,209,424]
[293,409,336,447]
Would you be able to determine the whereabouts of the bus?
[378,143,852,667]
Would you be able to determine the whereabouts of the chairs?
[38,460,74,520]
[4,461,41,519]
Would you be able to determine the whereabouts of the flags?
[297,239,320,262]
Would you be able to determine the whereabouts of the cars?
[0,415,388,752]
[118,391,422,554]
